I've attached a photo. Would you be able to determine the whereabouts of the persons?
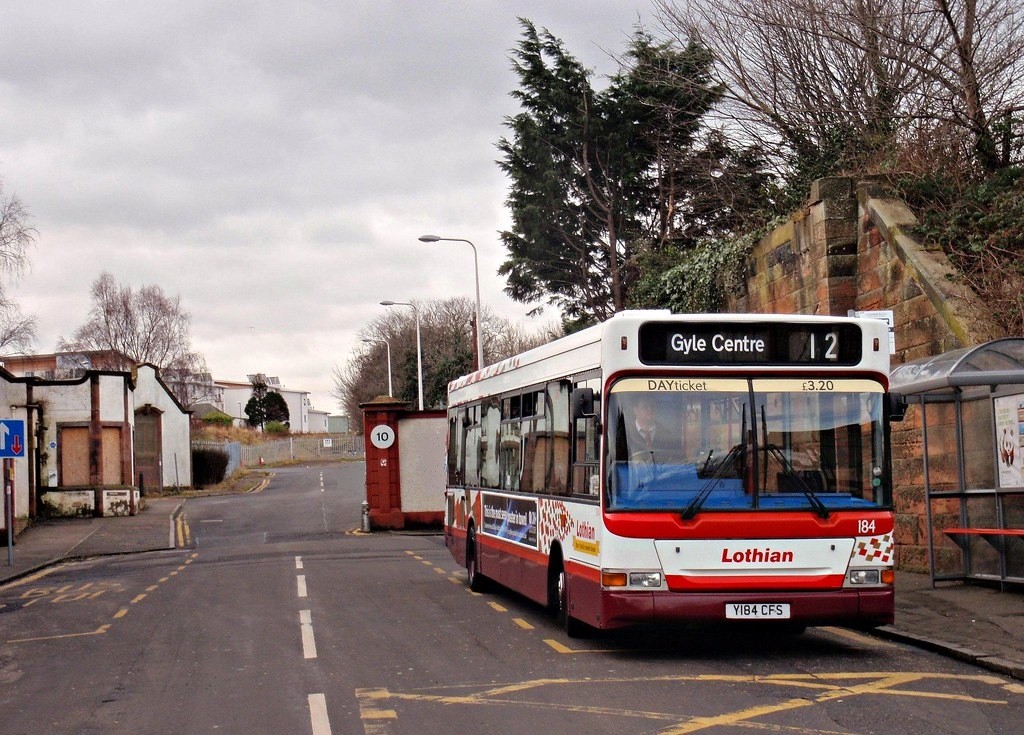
[628,397,677,452]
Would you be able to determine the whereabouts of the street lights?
[418,235,484,371]
[362,339,392,397]
[380,301,424,411]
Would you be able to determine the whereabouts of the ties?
[640,428,653,447]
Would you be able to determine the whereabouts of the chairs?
[777,471,825,494]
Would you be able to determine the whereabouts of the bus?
[446,310,908,641]
